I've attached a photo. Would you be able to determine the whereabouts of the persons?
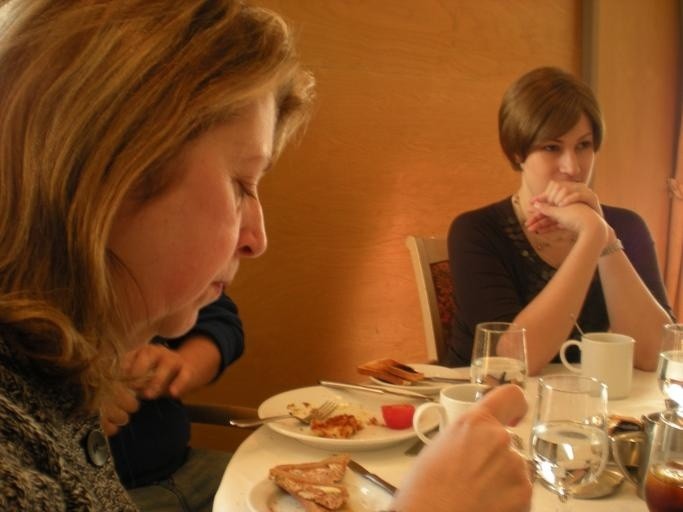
[95,286,246,512]
[0,1,533,512]
[448,63,681,381]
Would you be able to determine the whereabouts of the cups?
[412,384,495,446]
[643,410,683,512]
[560,333,636,401]
[656,323,683,409]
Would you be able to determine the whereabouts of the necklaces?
[513,196,574,251]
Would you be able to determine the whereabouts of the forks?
[229,399,339,428]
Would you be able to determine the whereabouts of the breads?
[268,456,353,512]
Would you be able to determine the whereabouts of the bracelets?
[594,238,624,260]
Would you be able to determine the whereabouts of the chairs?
[405,236,453,368]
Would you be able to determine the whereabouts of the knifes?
[424,377,470,384]
[317,378,434,403]
[334,454,399,497]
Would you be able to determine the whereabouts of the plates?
[258,385,441,453]
[370,363,460,395]
[247,471,393,511]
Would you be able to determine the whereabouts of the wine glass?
[470,322,528,450]
[531,375,610,512]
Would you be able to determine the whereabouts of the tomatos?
[383,403,415,430]
